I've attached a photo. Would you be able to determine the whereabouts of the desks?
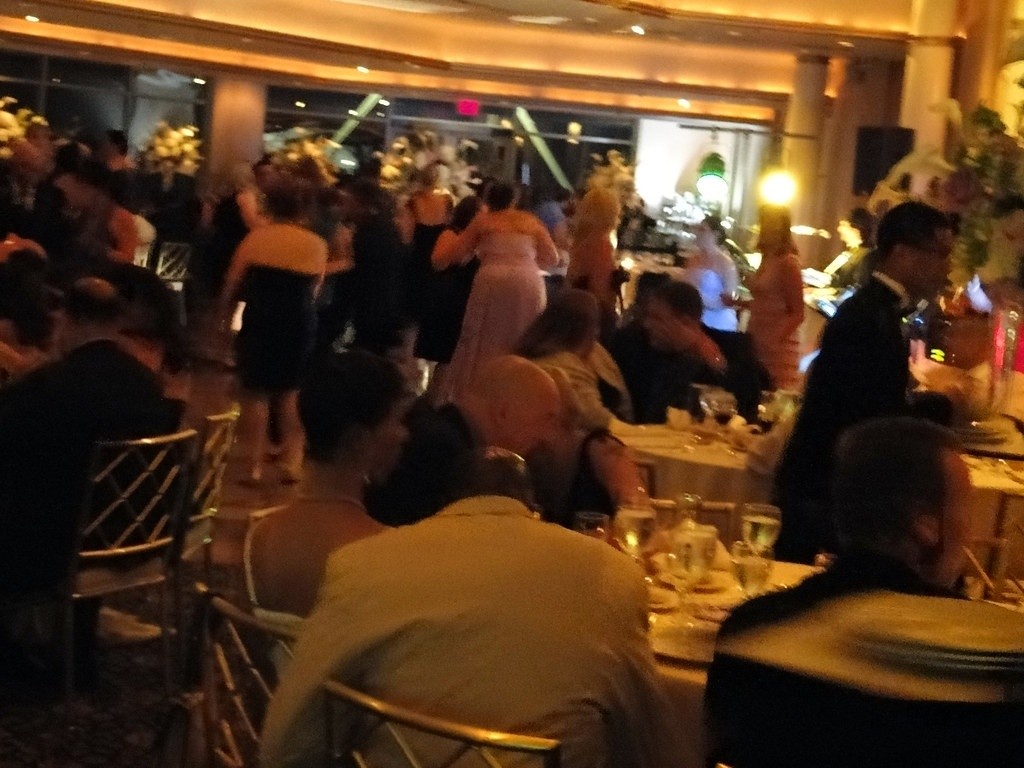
[611,424,1024,579]
[646,559,825,768]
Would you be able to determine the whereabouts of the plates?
[652,629,719,660]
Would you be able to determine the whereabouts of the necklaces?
[297,493,368,514]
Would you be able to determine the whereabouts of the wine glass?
[707,395,734,451]
[570,496,788,633]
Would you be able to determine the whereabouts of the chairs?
[0,403,563,768]
[130,242,193,327]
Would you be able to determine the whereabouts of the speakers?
[853,126,916,197]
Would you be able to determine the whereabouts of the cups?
[757,405,782,436]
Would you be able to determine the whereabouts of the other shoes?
[241,474,261,487]
[280,469,300,483]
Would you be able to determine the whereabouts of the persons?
[766,200,976,594]
[0,123,1024,768]
[705,418,1024,768]
